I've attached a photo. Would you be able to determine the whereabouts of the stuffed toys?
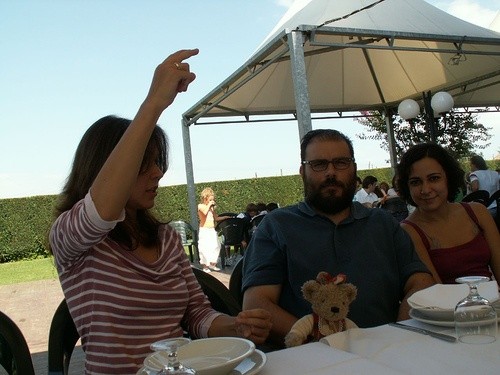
[285,272,358,347]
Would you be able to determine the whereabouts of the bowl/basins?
[144,337,255,375]
[407,280,499,320]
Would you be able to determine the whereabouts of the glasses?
[303,158,355,171]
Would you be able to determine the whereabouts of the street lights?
[398,90,455,144]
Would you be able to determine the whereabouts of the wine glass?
[453,276,499,344]
[149,337,197,375]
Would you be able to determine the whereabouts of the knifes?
[388,320,457,342]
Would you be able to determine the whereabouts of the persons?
[242,129,436,352]
[395,142,500,284]
[46,48,273,375]
[468,155,500,217]
[236,202,278,249]
[353,175,416,217]
[197,188,231,273]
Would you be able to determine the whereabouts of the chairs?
[169,220,201,264]
[217,219,247,269]
[49,269,258,375]
[488,190,500,233]
[376,197,408,222]
[461,190,490,206]
[0,310,35,375]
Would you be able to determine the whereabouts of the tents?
[180,0,500,268]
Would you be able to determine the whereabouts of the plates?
[410,308,499,326]
[136,348,267,375]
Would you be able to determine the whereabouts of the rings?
[175,63,179,67]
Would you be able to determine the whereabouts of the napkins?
[407,280,500,314]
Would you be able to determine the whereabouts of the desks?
[259,314,499,375]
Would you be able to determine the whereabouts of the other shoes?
[232,253,237,261]
[225,258,233,265]
[209,265,220,271]
[203,267,211,273]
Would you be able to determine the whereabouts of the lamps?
[397,90,454,142]
